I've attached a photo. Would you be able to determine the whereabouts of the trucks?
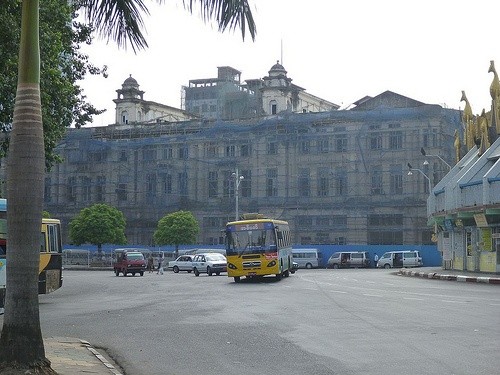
[114,250,146,276]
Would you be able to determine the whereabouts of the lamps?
[407,162,431,194]
[421,147,451,171]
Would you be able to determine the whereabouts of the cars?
[168,253,227,276]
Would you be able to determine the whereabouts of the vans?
[377,250,423,269]
[327,251,369,269]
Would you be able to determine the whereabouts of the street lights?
[232,168,244,221]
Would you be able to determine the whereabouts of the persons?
[395,255,400,265]
[156,254,163,275]
[374,253,378,268]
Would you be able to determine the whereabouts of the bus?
[0,199,63,307]
[292,249,323,269]
[225,219,292,282]
